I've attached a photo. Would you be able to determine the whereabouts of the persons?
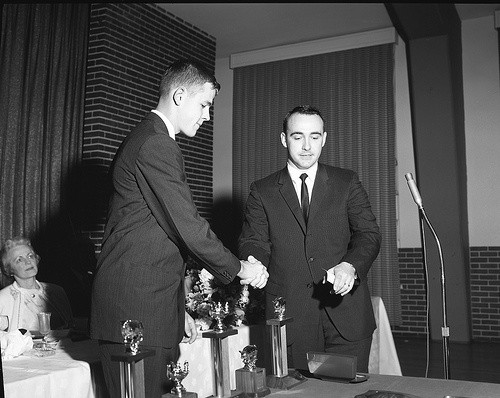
[0,237,73,332]
[236,105,382,375]
[88,59,270,398]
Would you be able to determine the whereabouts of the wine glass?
[38,313,51,351]
[0,315,10,356]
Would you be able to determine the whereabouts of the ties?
[299,173,310,225]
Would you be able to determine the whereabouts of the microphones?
[406,173,447,328]
[39,295,91,341]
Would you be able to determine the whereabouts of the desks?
[217,366,499,398]
[0,298,386,397]
